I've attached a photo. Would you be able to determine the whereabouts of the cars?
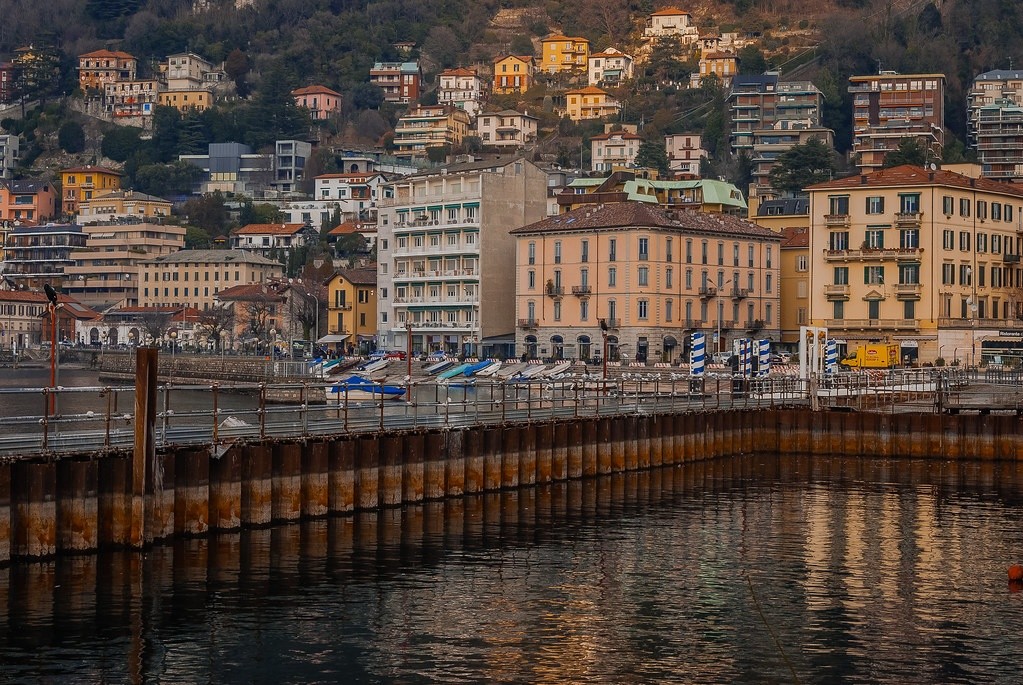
[685,349,809,365]
[386,350,406,361]
[369,350,389,359]
[426,350,454,361]
[404,351,428,362]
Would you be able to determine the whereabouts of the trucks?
[838,340,902,368]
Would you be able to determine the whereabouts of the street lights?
[269,329,277,363]
[221,330,227,365]
[464,287,474,358]
[147,333,152,350]
[171,332,177,358]
[127,333,133,365]
[306,292,319,342]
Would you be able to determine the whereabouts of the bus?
[281,340,307,358]
[303,341,327,358]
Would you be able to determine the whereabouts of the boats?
[422,360,454,376]
[435,363,473,380]
[323,375,406,400]
[476,363,501,376]
[514,365,547,379]
[466,359,492,372]
[540,361,573,376]
[309,356,344,376]
[493,362,527,380]
[351,358,389,374]
